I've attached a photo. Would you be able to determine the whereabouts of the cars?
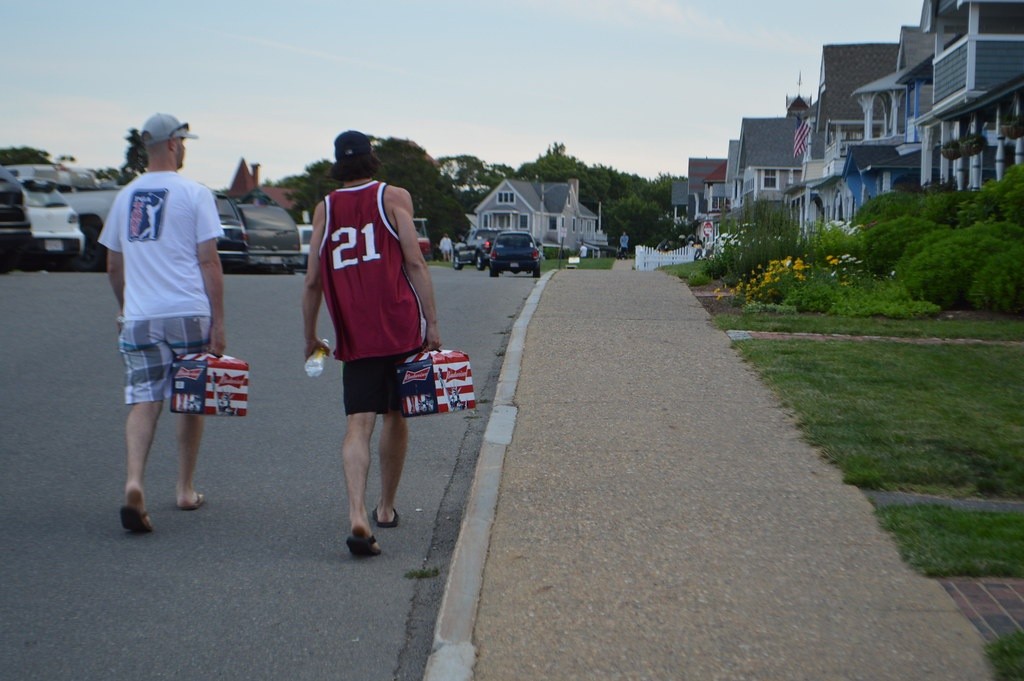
[0,165,34,274]
[15,180,84,270]
[452,228,506,271]
[237,203,300,272]
[487,231,541,278]
[297,222,315,255]
[415,232,430,256]
[214,192,249,273]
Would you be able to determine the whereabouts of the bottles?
[304,338,330,378]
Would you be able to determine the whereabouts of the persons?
[439,230,464,262]
[301,131,444,556]
[107,113,226,533]
[576,242,588,258]
[617,230,631,260]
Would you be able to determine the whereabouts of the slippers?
[176,492,207,510]
[372,505,400,528]
[346,534,381,556]
[120,506,153,532]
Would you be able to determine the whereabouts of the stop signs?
[703,221,713,236]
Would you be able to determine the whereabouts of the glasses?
[169,123,189,137]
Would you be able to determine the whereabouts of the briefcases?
[396,344,476,417]
[170,346,249,416]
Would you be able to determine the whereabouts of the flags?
[794,116,811,160]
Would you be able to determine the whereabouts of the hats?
[141,112,198,146]
[334,130,373,160]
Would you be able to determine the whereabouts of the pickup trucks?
[10,165,125,272]
